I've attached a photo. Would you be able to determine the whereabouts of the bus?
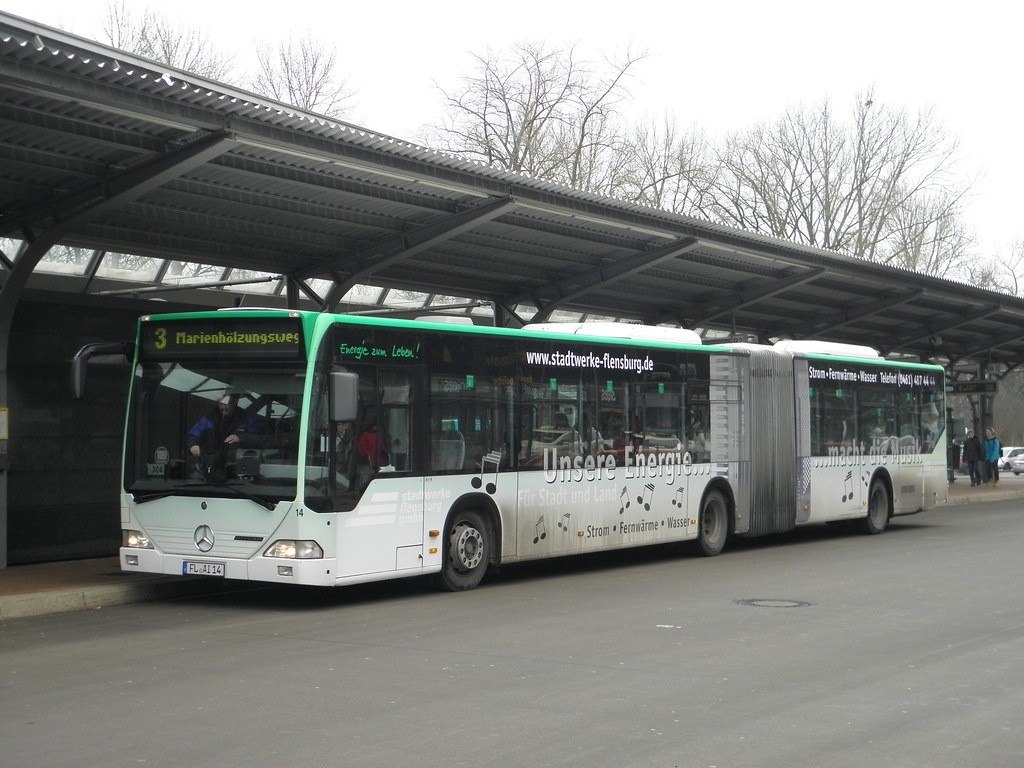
[66,305,949,594]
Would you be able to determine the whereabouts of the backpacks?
[993,439,1003,457]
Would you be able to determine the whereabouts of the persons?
[982,427,1000,486]
[187,391,255,457]
[910,395,939,454]
[334,422,387,489]
[962,431,984,487]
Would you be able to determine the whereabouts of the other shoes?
[970,483,975,486]
[993,480,999,486]
[977,481,981,485]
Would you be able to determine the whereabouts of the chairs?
[816,404,939,448]
[427,406,469,475]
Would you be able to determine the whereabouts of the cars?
[997,446,1024,472]
[1010,452,1024,476]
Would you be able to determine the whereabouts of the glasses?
[217,402,233,406]
